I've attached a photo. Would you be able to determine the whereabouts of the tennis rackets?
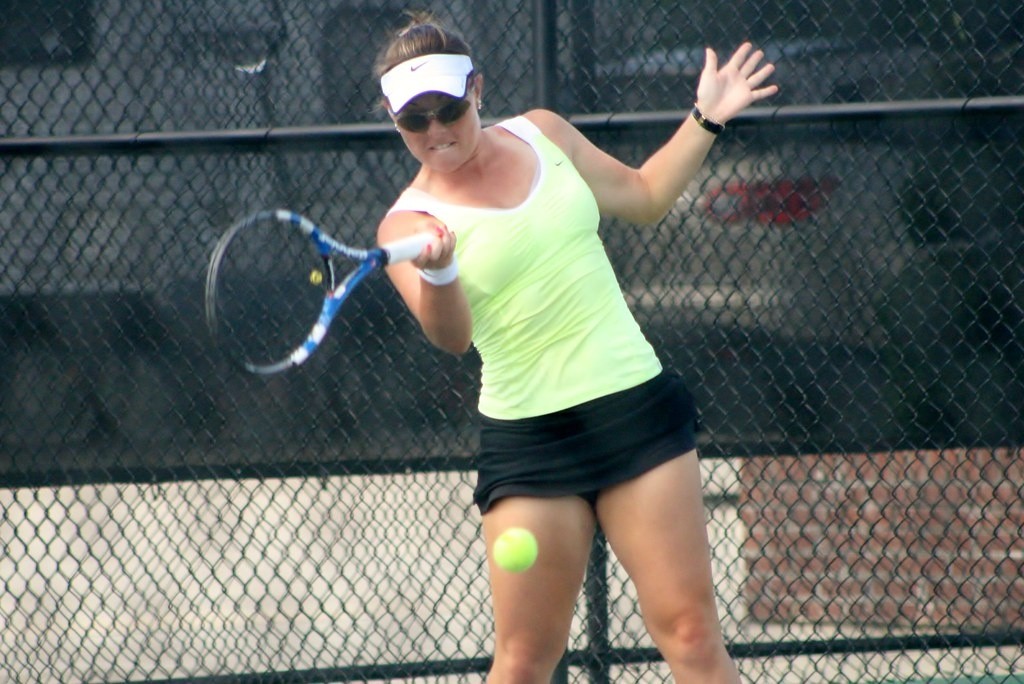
[203,206,455,377]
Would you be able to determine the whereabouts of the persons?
[373,22,778,684]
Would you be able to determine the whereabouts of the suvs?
[573,38,1024,442]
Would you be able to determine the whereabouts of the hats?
[380,53,473,116]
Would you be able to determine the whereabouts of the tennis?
[491,526,541,573]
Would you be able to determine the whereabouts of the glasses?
[386,84,476,133]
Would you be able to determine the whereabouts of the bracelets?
[418,255,460,286]
[692,102,726,135]
